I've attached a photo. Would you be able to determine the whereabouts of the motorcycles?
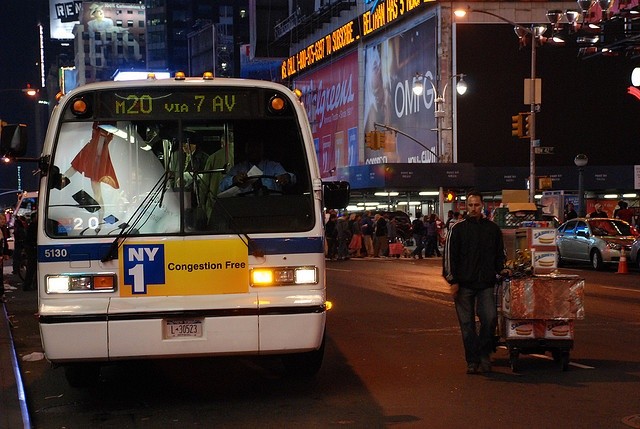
[18,246,37,290]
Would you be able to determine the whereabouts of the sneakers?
[478,354,491,375]
[466,362,478,374]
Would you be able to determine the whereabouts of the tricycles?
[494,249,586,372]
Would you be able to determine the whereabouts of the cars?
[6,190,39,251]
[628,237,640,272]
[555,216,640,272]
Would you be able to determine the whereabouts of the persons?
[586,202,609,218]
[441,193,506,375]
[564,203,576,224]
[171,136,208,185]
[61,120,120,232]
[217,140,288,192]
[614,199,636,223]
[199,134,234,219]
[1,209,38,297]
[325,207,462,260]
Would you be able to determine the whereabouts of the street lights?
[21,87,44,168]
[453,5,548,211]
[371,70,469,238]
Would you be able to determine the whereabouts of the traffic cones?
[614,244,631,275]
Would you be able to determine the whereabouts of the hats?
[371,61,383,95]
[595,203,602,208]
[89,3,103,18]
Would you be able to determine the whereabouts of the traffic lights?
[375,131,385,150]
[365,133,375,150]
[511,111,532,139]
[525,111,536,138]
[447,189,455,203]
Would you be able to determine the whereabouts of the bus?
[1,71,351,388]
